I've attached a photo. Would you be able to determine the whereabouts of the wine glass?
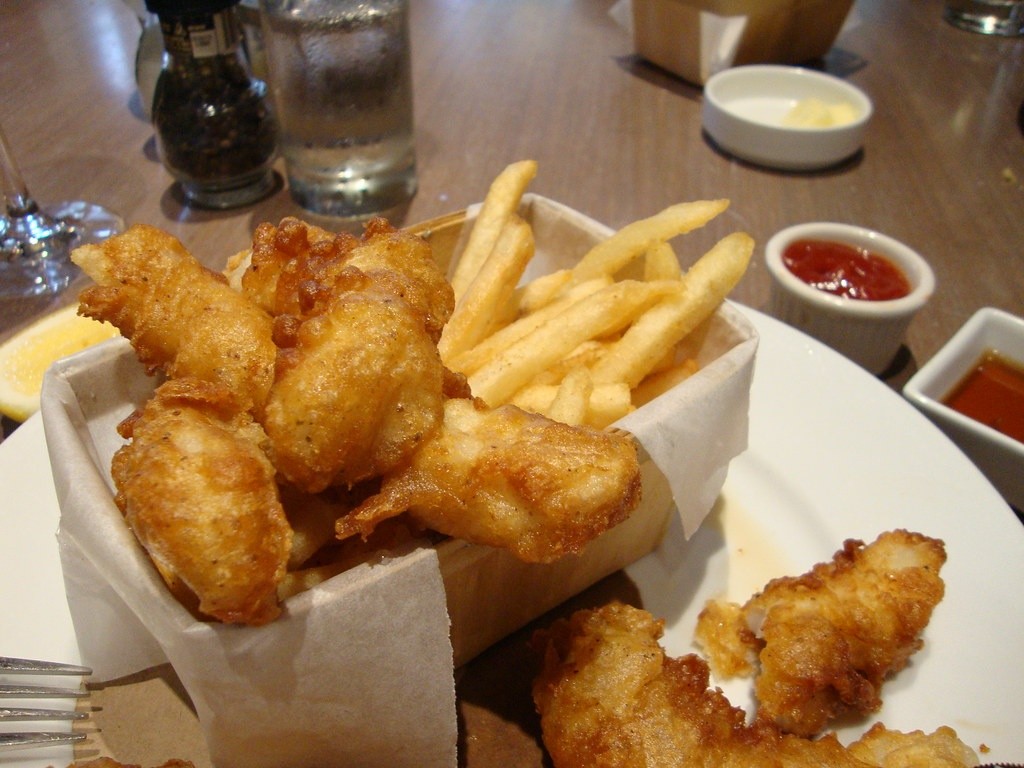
[1,118,126,302]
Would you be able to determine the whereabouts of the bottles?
[141,0,281,205]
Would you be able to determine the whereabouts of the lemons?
[0,302,120,423]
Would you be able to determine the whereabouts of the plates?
[907,305,1023,520]
[702,63,873,172]
[0,269,1023,767]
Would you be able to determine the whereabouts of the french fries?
[435,159,755,428]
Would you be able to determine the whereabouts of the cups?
[251,0,419,217]
[941,0,1024,37]
[765,219,936,380]
